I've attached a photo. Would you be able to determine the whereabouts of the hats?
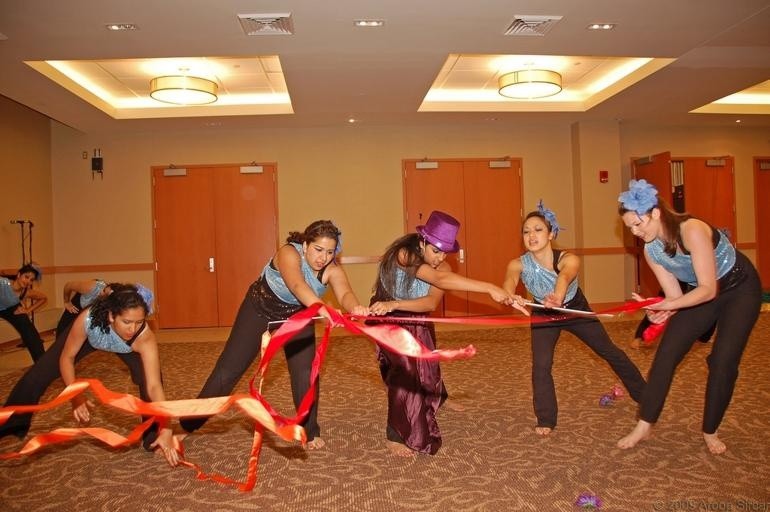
[416,211,460,253]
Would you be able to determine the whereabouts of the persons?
[0,260,48,364]
[628,279,719,351]
[364,210,517,455]
[154,218,372,460]
[0,284,182,469]
[612,178,764,456]
[503,199,647,439]
[57,278,125,341]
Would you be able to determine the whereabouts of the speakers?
[92,157,103,171]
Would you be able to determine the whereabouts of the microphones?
[10,219,29,224]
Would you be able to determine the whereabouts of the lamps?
[147,73,222,107]
[496,69,564,99]
